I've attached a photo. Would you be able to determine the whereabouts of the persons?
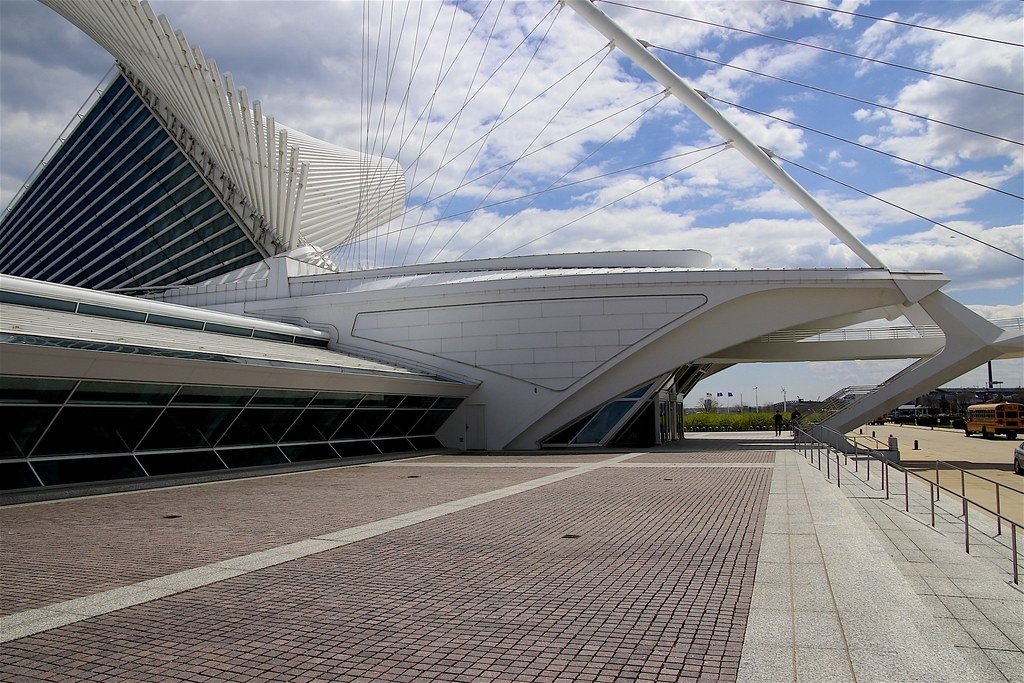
[773,410,783,436]
[791,408,802,440]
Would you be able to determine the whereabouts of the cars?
[1013,441,1024,476]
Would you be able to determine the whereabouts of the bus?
[867,412,887,425]
[964,402,1024,440]
